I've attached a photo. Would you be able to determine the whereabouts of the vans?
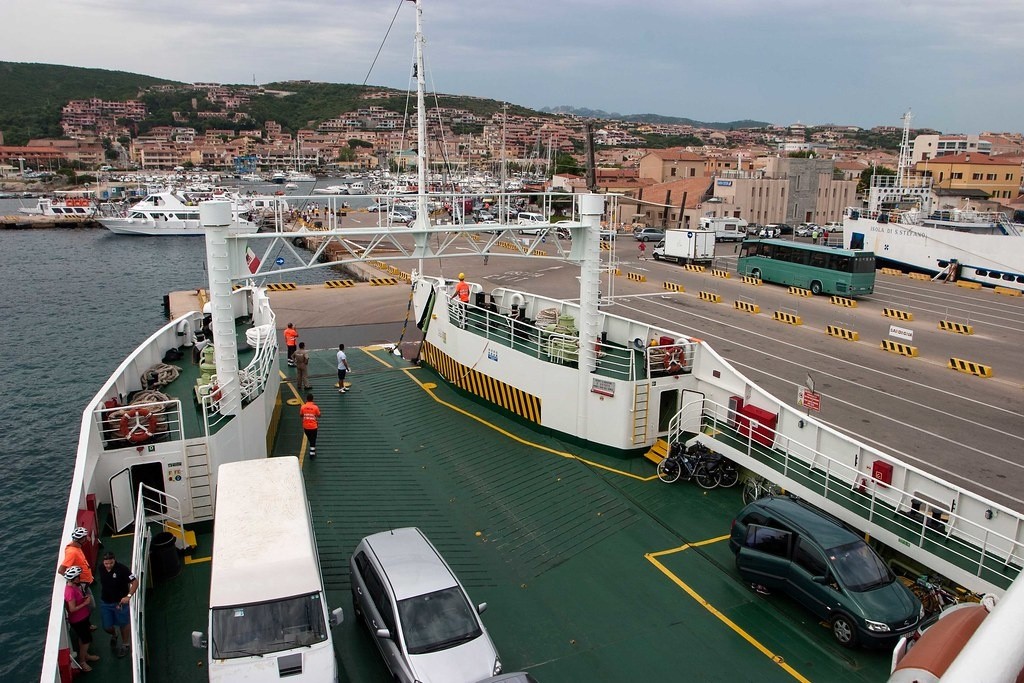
[191,455,344,683]
[517,212,552,236]
[447,199,472,216]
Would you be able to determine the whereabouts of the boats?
[842,201,1024,295]
[92,191,259,237]
[0,99,558,216]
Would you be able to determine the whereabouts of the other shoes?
[109,631,118,650]
[304,386,313,390]
[339,388,345,393]
[343,386,350,391]
[309,453,316,461]
[298,386,302,389]
[119,642,131,656]
[86,655,99,660]
[80,662,92,672]
[288,359,297,368]
[90,625,97,630]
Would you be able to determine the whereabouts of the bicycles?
[910,570,985,622]
[741,474,775,506]
[657,441,738,490]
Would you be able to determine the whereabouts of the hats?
[288,323,293,327]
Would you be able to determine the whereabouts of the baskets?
[669,446,686,458]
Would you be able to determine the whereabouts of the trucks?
[652,228,716,267]
[700,216,749,243]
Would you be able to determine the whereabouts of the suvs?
[728,496,925,649]
[758,225,781,239]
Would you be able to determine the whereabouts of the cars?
[389,204,437,220]
[824,222,843,233]
[769,223,793,235]
[388,210,413,223]
[366,202,391,212]
[634,227,665,242]
[472,210,494,222]
[478,221,503,234]
[795,222,824,237]
[747,223,764,236]
[350,527,502,683]
[489,202,526,219]
[553,219,618,240]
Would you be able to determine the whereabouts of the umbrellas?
[100,165,112,174]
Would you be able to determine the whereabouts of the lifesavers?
[510,293,526,308]
[663,348,685,371]
[120,407,158,442]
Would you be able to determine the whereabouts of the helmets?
[458,273,466,280]
[72,527,88,539]
[63,565,82,579]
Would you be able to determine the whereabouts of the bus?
[733,238,876,298]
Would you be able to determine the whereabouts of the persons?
[336,344,351,393]
[181,197,213,206]
[450,273,470,330]
[636,241,648,261]
[56,195,83,202]
[59,527,139,672]
[764,228,768,239]
[824,230,829,241]
[284,323,314,393]
[304,200,350,227]
[300,394,320,457]
[812,230,819,245]
